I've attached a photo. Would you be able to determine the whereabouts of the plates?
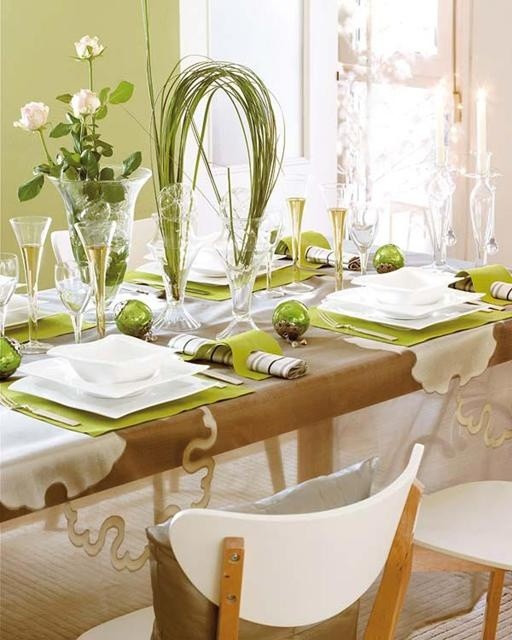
[319,282,488,330]
[5,354,221,421]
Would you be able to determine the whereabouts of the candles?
[476,89,488,172]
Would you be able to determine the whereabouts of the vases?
[46,165,152,324]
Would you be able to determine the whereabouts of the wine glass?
[276,172,317,295]
[54,217,121,347]
[207,213,272,344]
[7,214,57,355]
[321,182,352,291]
[150,211,203,333]
[347,198,380,282]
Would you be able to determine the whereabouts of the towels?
[167,332,309,379]
[449,270,512,303]
[303,243,364,272]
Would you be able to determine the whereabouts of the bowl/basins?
[356,265,463,305]
[45,333,176,385]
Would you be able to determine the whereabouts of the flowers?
[12,35,143,288]
